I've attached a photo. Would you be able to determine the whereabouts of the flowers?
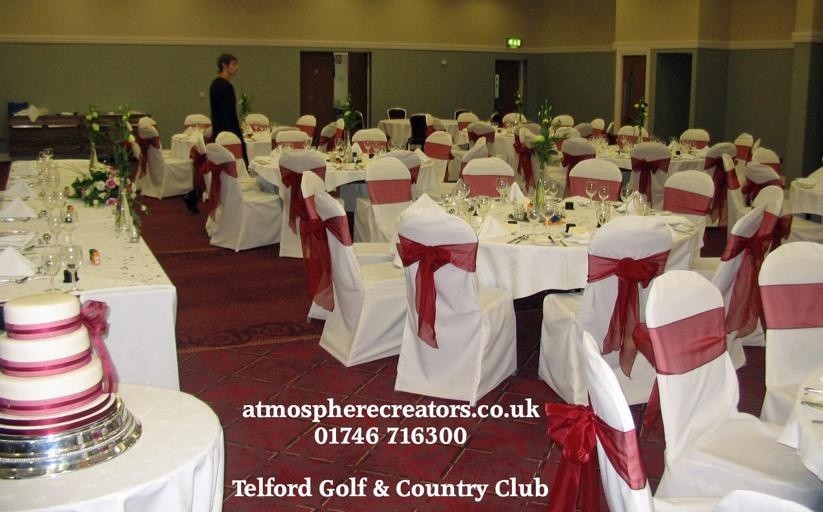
[64,159,152,243]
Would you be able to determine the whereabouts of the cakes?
[0,292,116,434]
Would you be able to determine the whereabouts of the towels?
[1,197,36,220]
[0,243,34,277]
[1,231,36,248]
[1,177,35,198]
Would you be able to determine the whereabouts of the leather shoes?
[183,195,201,213]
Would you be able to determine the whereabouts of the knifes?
[548,235,555,246]
[507,236,521,244]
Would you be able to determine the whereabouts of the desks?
[7,114,152,159]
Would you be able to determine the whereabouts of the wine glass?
[36,147,82,294]
[487,120,518,135]
[277,137,406,168]
[588,134,693,164]
[253,120,276,136]
[442,178,648,235]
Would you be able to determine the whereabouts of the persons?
[209,52,249,176]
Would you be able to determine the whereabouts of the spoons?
[516,235,529,243]
[1,217,30,222]
[0,195,30,202]
[1,276,28,283]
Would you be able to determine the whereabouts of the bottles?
[89,142,97,169]
[117,176,127,235]
